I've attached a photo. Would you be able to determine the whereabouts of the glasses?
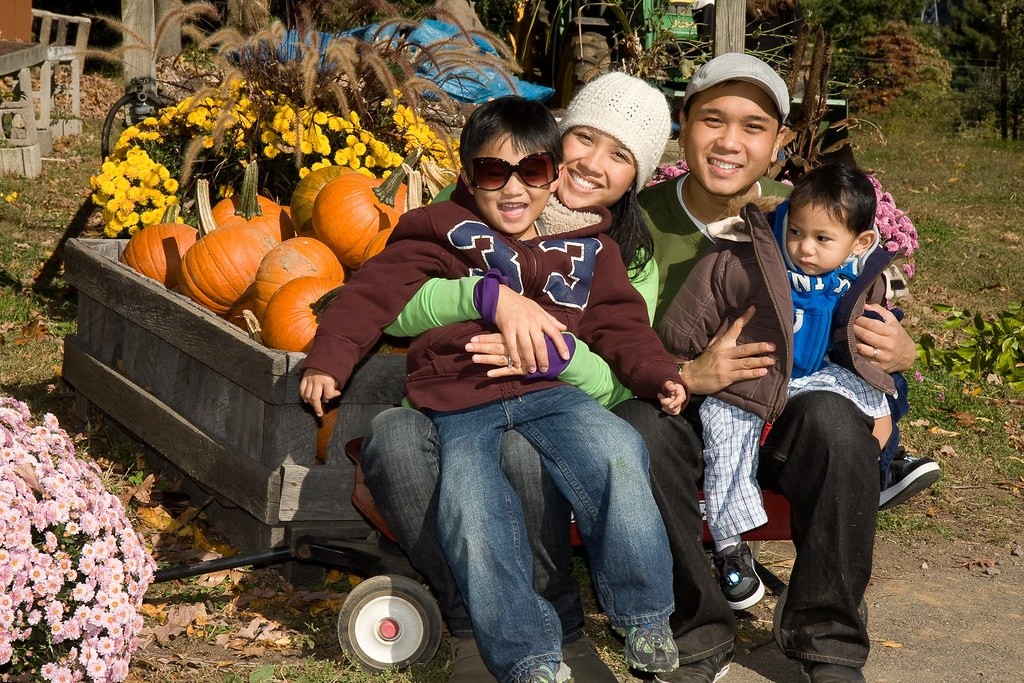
[465,151,561,192]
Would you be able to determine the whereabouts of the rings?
[872,348,877,357]
[509,356,513,365]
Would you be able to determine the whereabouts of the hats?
[561,72,670,194]
[683,53,791,126]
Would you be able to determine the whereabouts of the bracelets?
[677,364,683,375]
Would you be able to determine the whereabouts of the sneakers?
[710,543,764,611]
[654,643,736,683]
[793,648,866,683]
[512,653,574,683]
[613,616,678,672]
[878,444,940,512]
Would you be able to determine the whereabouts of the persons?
[364,72,670,683]
[638,53,914,683]
[657,163,940,611]
[300,95,689,683]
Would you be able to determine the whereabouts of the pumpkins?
[119,168,438,463]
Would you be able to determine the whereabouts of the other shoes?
[557,619,585,643]
[439,611,474,638]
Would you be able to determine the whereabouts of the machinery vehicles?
[508,0,848,140]
